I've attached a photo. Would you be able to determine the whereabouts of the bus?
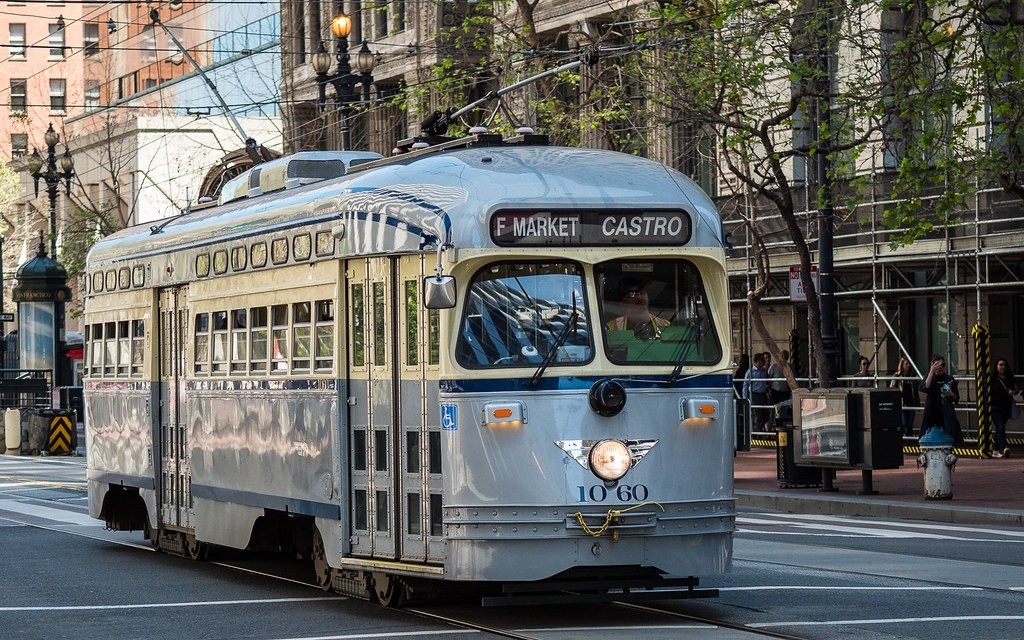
[82,51,752,608]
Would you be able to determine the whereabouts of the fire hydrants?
[916,425,958,502]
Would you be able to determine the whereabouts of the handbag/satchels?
[1010,399,1018,421]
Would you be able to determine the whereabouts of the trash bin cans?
[775,426,837,489]
[793,387,907,472]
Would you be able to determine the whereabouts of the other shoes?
[992,451,1003,458]
[753,435,760,440]
[767,437,774,441]
[1003,448,1011,458]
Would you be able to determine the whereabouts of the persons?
[851,356,875,388]
[990,358,1019,459]
[605,276,673,330]
[734,350,798,441]
[890,355,921,438]
[918,355,966,448]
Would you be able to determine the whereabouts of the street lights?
[311,1,375,149]
[27,121,78,410]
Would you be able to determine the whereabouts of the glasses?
[863,362,870,366]
[627,288,647,298]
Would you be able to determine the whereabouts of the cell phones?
[940,363,945,367]
